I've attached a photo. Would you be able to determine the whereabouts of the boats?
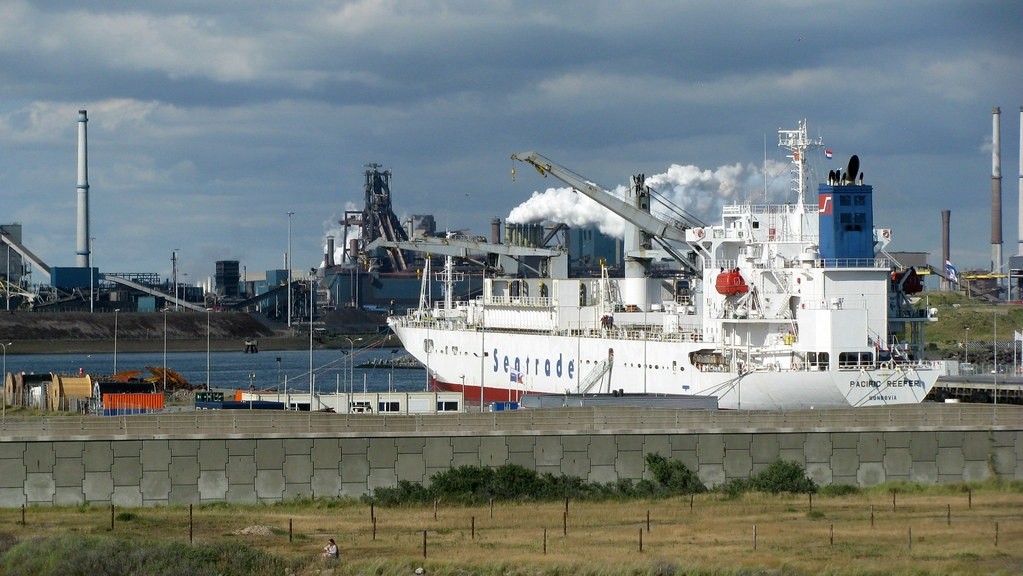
[367,118,944,407]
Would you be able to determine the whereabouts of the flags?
[824,149,833,159]
[945,258,958,282]
[509,366,524,385]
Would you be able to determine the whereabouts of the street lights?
[205,308,213,391]
[90,238,96,313]
[346,336,363,413]
[163,307,170,390]
[0,342,12,424]
[287,211,295,327]
[113,309,121,375]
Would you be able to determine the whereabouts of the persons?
[322,539,337,558]
